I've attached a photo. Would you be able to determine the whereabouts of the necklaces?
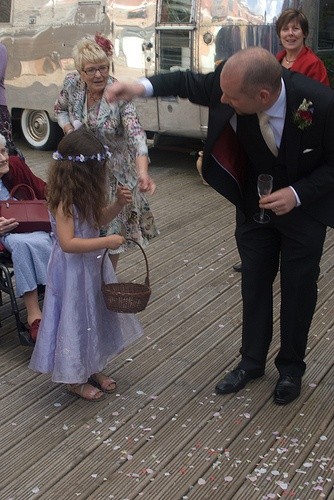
[286,55,295,64]
[87,87,102,102]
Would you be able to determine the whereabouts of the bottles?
[196,151,210,185]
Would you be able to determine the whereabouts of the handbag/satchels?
[0,184,53,233]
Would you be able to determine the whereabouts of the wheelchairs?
[0,250,48,347]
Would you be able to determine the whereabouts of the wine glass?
[254,173,273,222]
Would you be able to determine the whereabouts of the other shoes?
[29,319,41,341]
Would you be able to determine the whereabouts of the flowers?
[51,144,113,163]
[292,98,315,130]
[93,33,114,57]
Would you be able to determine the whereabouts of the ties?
[258,113,278,156]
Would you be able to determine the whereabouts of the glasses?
[84,65,109,75]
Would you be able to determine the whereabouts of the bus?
[0,1,334,188]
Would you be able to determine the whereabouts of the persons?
[233,9,330,272]
[0,44,18,156]
[0,134,53,344]
[103,47,334,405]
[54,36,160,272]
[28,129,144,402]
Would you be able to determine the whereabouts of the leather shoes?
[274,376,301,404]
[215,369,251,395]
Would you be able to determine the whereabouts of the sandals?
[90,373,117,394]
[67,383,104,402]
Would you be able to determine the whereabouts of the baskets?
[101,239,151,314]
